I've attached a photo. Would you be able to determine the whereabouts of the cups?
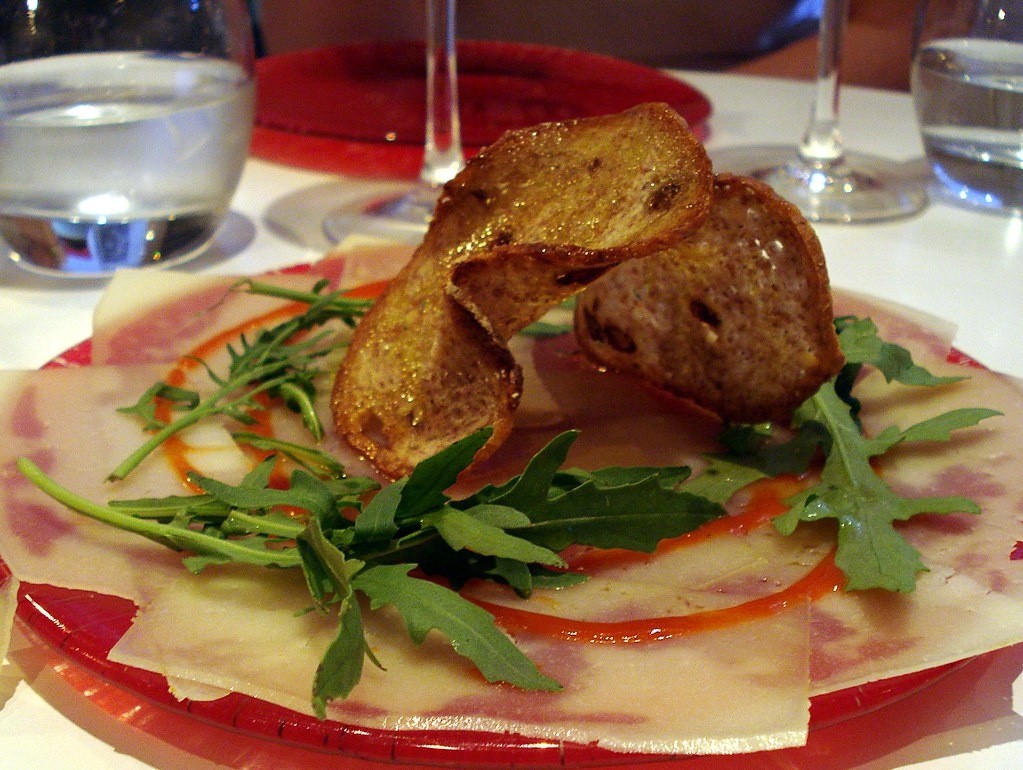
[912,0,1022,211]
[2,1,255,281]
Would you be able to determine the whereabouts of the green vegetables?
[17,275,1007,724]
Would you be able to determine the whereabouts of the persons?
[0,0,230,67]
[219,0,974,93]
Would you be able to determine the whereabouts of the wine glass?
[321,3,466,239]
[706,0,928,224]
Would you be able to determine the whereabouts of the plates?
[0,246,1022,766]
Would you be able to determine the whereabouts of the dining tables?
[0,63,1023,770]
